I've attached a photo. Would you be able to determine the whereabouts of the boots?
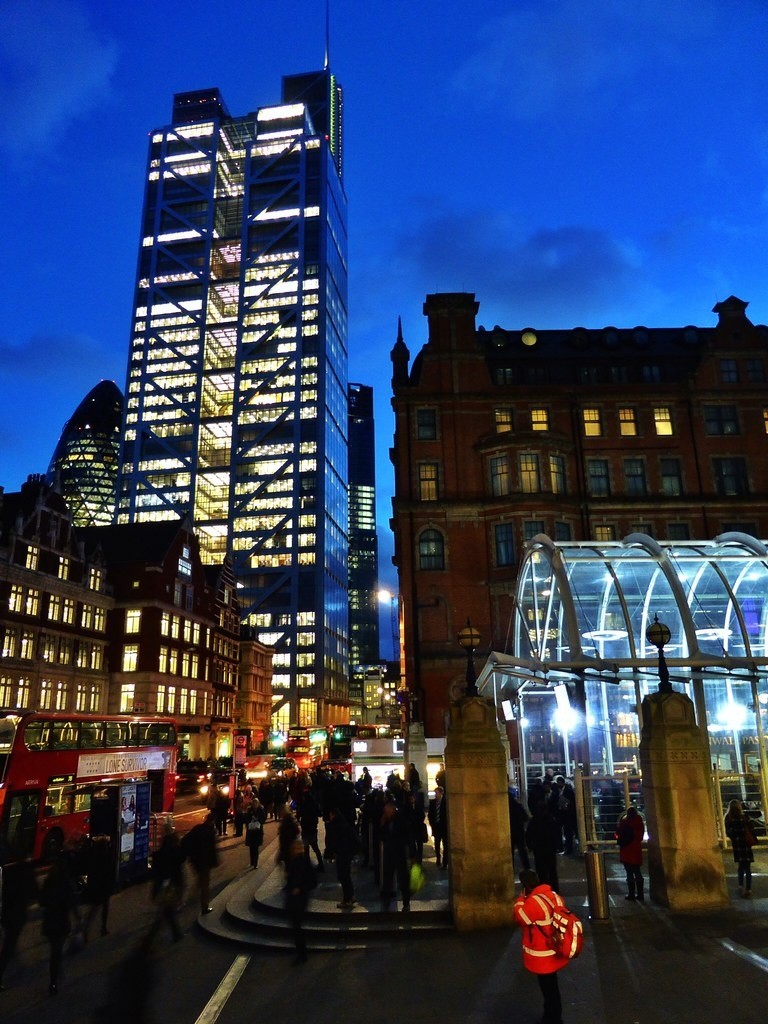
[625,878,645,900]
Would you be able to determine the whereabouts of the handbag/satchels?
[617,821,633,847]
[248,819,261,830]
[409,864,425,894]
[744,813,758,847]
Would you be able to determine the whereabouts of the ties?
[436,801,440,823]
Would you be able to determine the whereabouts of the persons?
[514,868,565,1024]
[121,797,127,811]
[615,807,646,902]
[0,748,447,994]
[725,799,757,897]
[129,795,134,811]
[507,767,641,893]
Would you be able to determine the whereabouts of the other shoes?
[736,887,753,898]
[402,905,410,913]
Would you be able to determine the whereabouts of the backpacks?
[538,892,585,959]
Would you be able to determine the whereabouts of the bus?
[0,709,180,876]
[286,724,330,769]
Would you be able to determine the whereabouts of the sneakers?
[337,896,357,908]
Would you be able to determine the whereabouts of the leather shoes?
[202,907,213,915]
